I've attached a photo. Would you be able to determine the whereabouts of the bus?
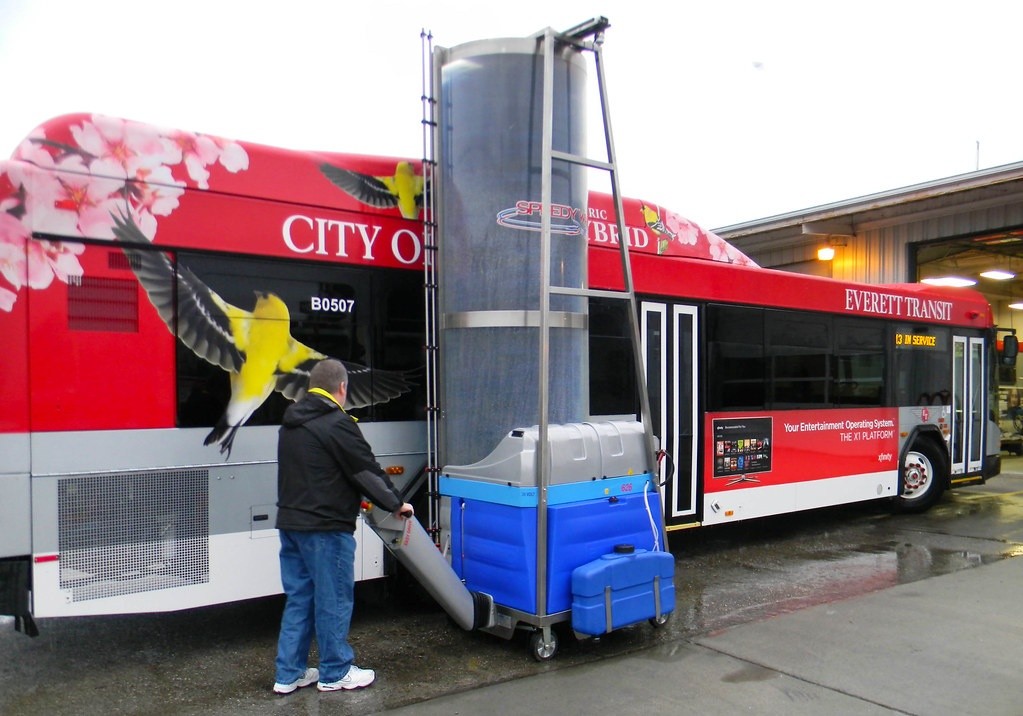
[1,114,1001,637]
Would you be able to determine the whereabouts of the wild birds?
[639,205,677,241]
[318,161,430,221]
[108,197,425,463]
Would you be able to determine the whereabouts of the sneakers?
[316,663,375,692]
[272,667,320,694]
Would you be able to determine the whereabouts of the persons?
[270,357,415,694]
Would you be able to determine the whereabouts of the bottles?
[571,544,677,635]
